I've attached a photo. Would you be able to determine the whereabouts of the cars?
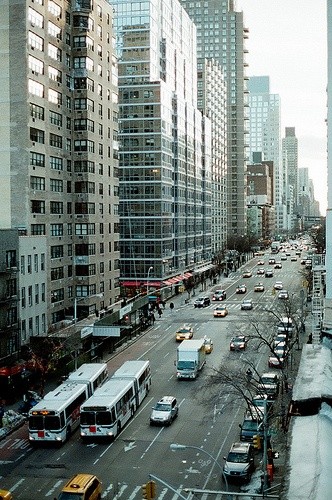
[229,335,248,351]
[268,318,298,368]
[203,336,214,354]
[191,228,313,317]
[175,327,192,341]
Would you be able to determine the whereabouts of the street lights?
[147,266,153,296]
[170,443,229,492]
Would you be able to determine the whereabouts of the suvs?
[221,441,254,483]
[246,395,272,420]
[255,371,282,400]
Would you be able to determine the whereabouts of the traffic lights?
[141,482,148,500]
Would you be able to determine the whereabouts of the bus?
[78,360,152,446]
[27,362,110,448]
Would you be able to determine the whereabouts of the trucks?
[175,338,207,381]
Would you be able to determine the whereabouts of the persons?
[170,301,174,312]
[158,309,163,318]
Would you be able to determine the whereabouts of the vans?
[150,395,178,426]
[238,417,268,451]
[55,474,102,500]
